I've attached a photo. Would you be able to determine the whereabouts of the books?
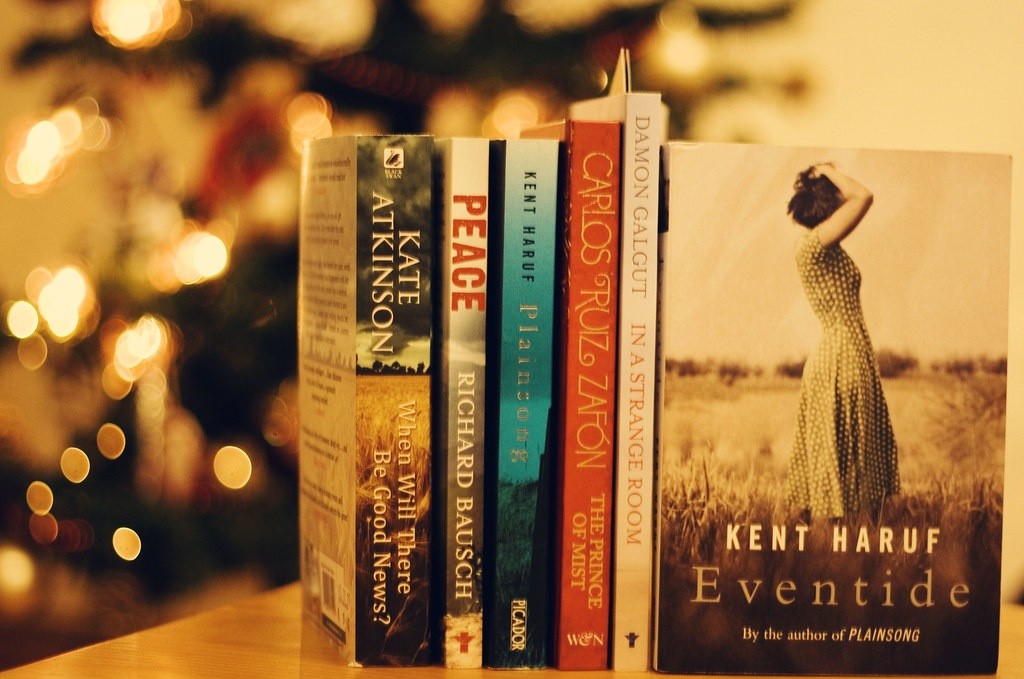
[297,45,1012,674]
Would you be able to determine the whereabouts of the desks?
[1,581,1024,679]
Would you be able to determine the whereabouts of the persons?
[787,160,903,526]
